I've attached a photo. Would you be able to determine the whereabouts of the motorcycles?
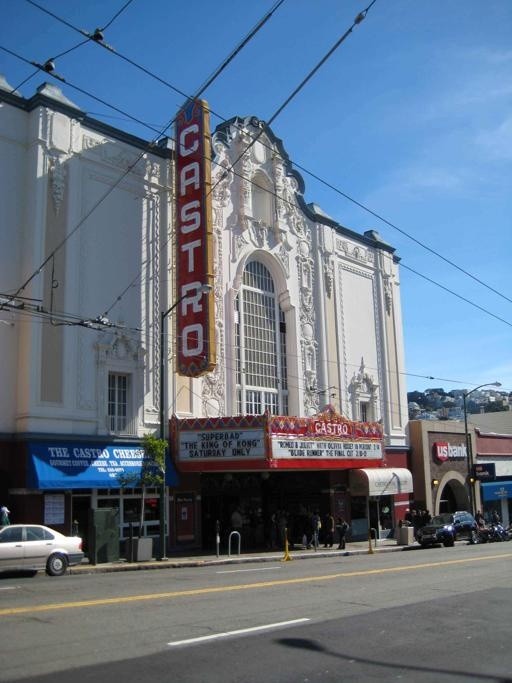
[480,522,512,543]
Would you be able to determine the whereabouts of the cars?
[0,524,85,576]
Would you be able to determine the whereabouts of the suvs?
[416,510,480,547]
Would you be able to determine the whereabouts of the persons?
[404,507,432,537]
[476,510,484,528]
[230,502,350,550]
[495,512,501,523]
[493,509,497,522]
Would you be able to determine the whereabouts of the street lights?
[463,380,502,519]
[157,284,214,562]
